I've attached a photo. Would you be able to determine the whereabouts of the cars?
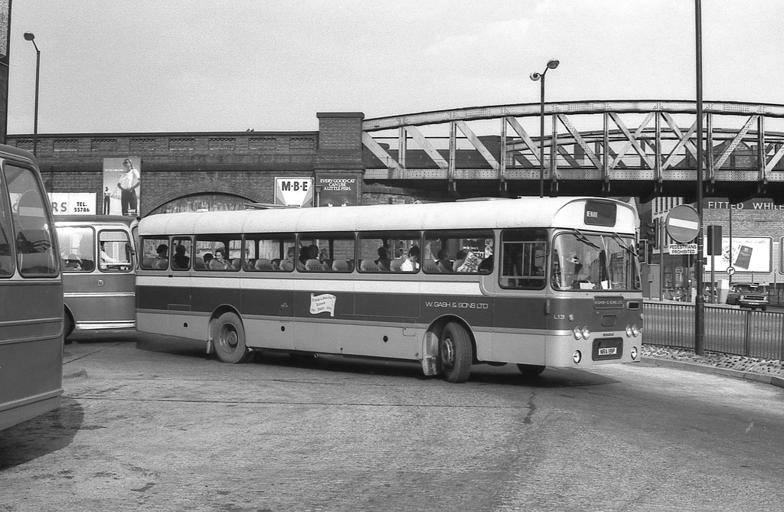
[727,283,768,313]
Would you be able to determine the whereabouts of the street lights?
[24,31,40,152]
[530,60,559,196]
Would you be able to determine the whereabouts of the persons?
[209,247,235,270]
[116,157,140,217]
[150,244,169,270]
[279,246,303,270]
[102,186,112,217]
[174,244,188,268]
[98,242,123,269]
[299,245,317,271]
[398,245,419,272]
[436,250,453,272]
[376,246,390,272]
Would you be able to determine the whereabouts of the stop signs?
[664,204,702,243]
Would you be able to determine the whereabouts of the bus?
[0,143,63,431]
